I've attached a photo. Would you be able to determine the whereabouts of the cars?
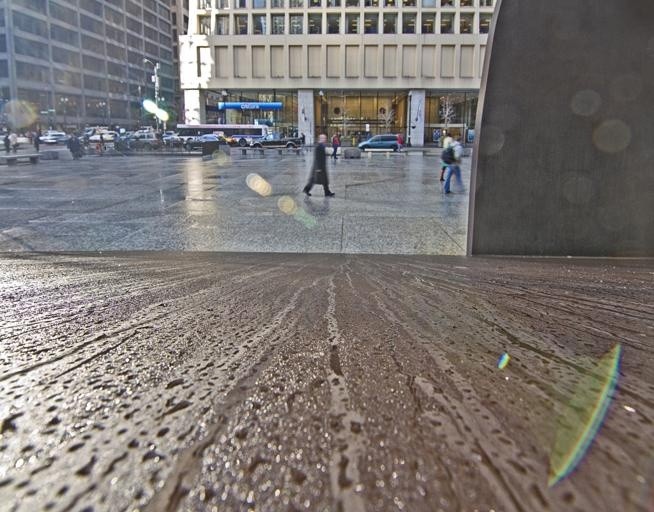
[358,134,400,152]
[0,126,227,148]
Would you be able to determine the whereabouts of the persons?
[397,133,403,153]
[12,142,19,152]
[3,135,10,153]
[444,135,466,194]
[114,134,120,148]
[330,133,340,159]
[84,134,89,146]
[439,133,454,181]
[100,134,103,144]
[302,134,335,196]
[34,136,40,152]
[300,133,306,146]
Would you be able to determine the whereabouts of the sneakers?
[445,191,451,193]
[440,178,445,181]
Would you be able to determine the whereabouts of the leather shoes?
[325,192,335,196]
[303,189,311,196]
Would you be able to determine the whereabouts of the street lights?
[143,58,161,132]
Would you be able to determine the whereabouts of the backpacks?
[441,143,462,164]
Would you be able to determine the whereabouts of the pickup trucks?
[250,130,302,148]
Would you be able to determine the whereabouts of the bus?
[176,123,266,147]
